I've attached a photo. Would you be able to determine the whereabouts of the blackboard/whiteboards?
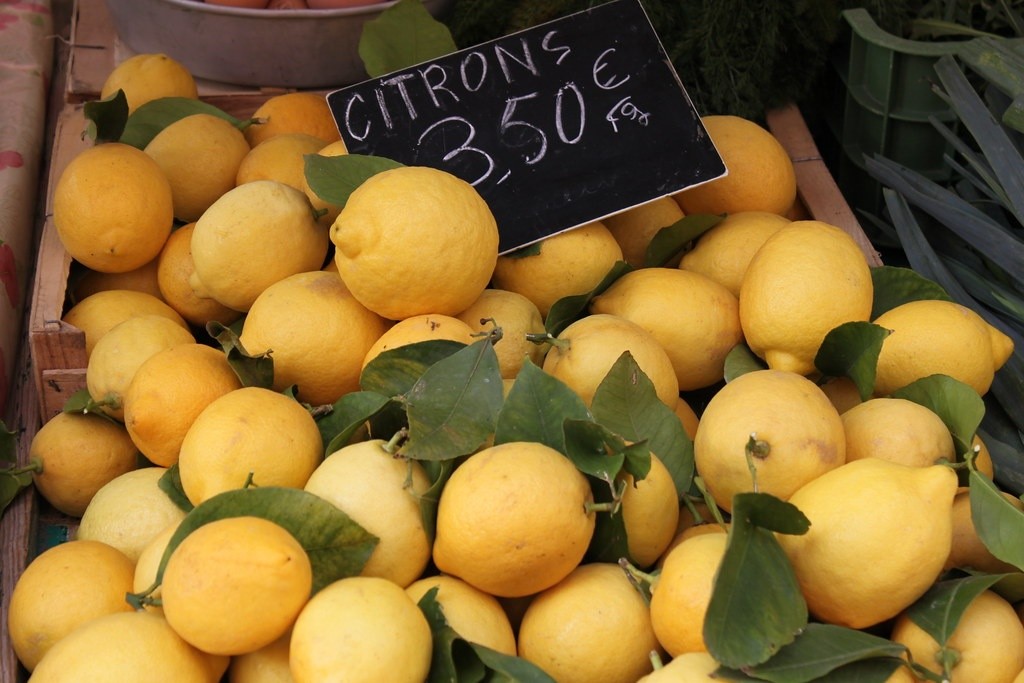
[325,0,729,258]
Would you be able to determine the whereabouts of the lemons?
[10,52,1024,683]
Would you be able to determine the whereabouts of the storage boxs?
[0,0,883,683]
